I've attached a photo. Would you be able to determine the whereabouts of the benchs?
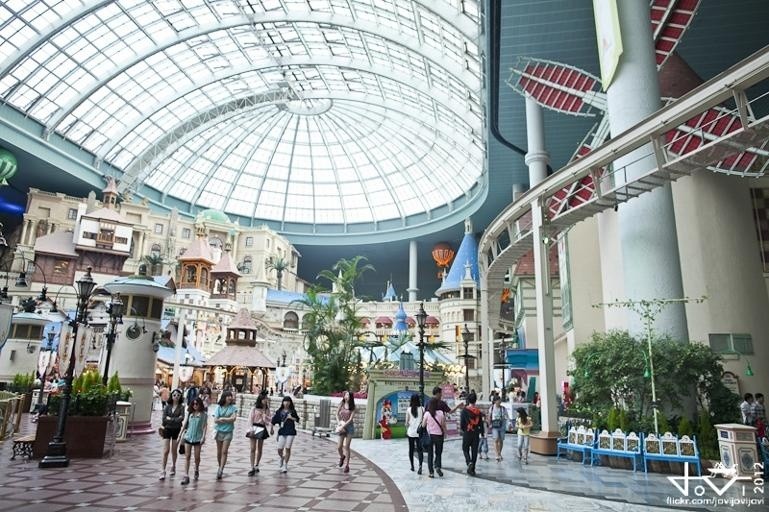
[587,425,643,474]
[310,425,333,439]
[555,422,600,468]
[642,430,701,478]
[10,431,39,461]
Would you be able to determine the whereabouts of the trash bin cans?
[115,400,132,442]
[715,424,761,481]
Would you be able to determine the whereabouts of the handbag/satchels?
[210,428,218,441]
[334,420,347,435]
[441,429,446,440]
[472,423,484,435]
[275,427,280,442]
[178,429,188,455]
[491,418,502,429]
[158,426,169,439]
[245,424,270,440]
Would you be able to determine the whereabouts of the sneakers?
[436,466,444,477]
[247,465,261,477]
[478,452,530,465]
[282,462,288,473]
[417,466,423,475]
[216,467,224,479]
[168,464,176,476]
[278,454,286,468]
[343,464,350,474]
[427,472,435,478]
[337,454,346,468]
[466,461,477,476]
[193,468,200,480]
[180,475,191,485]
[158,471,167,480]
[410,465,415,471]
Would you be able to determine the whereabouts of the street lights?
[457,324,478,401]
[415,302,430,406]
[492,336,513,395]
[37,266,97,470]
[715,346,754,379]
[0,238,49,303]
[102,291,126,385]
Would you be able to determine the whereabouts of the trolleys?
[312,404,332,439]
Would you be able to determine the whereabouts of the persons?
[150,383,274,411]
[750,393,765,426]
[245,392,274,476]
[176,397,207,485]
[270,396,300,473]
[335,391,356,473]
[159,389,185,480]
[405,386,541,478]
[740,393,754,426]
[33,373,65,390]
[212,391,238,480]
